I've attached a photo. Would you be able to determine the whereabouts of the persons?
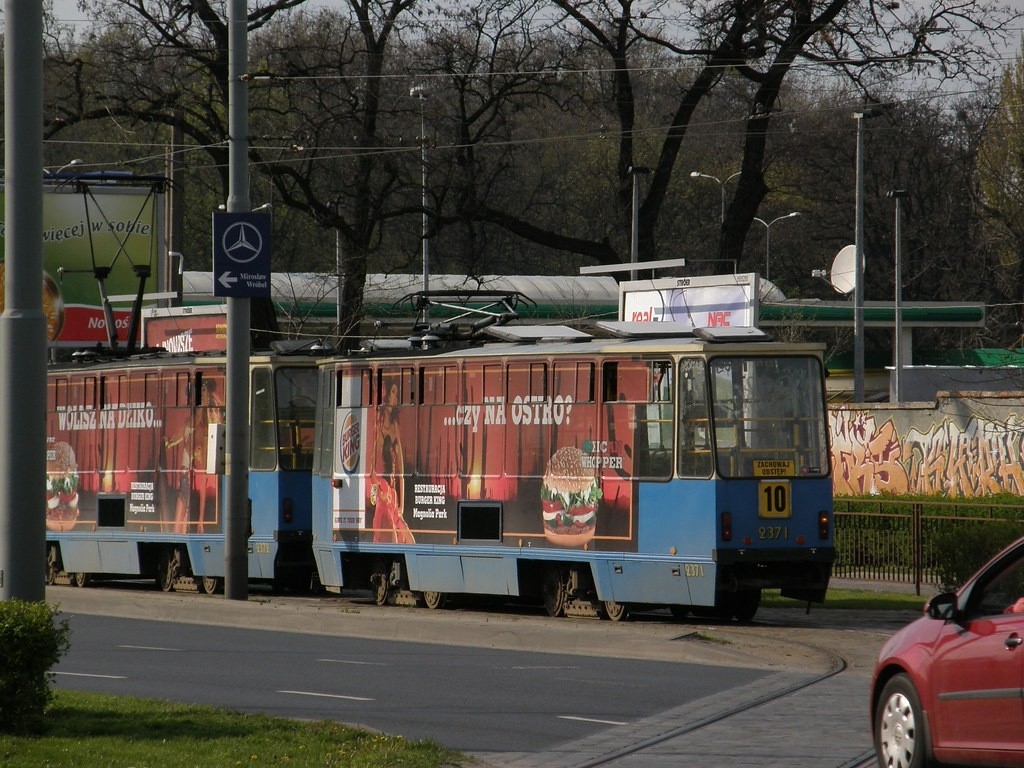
[367,367,404,544]
[168,376,223,534]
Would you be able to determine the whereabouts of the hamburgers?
[44,441,80,531]
[540,445,602,546]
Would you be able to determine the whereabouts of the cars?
[867,537,1024,768]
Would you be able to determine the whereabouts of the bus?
[40,154,838,623]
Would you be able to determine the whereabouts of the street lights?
[887,188,911,402]
[406,87,433,324]
[688,169,743,222]
[849,107,886,402]
[751,211,804,282]
[627,164,649,282]
